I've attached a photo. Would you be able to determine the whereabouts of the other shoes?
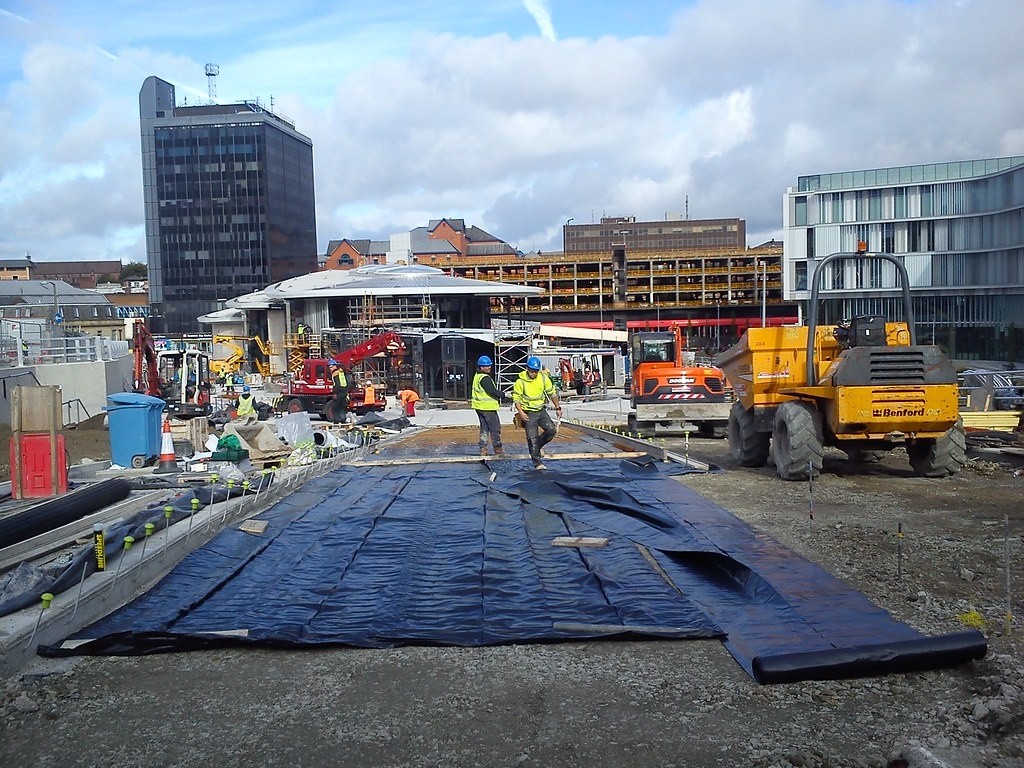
[407,414,415,417]
[540,447,545,458]
[536,463,546,471]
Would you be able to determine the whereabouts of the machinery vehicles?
[131,318,213,417]
[206,333,276,385]
[713,242,968,484]
[272,331,406,422]
[622,321,736,439]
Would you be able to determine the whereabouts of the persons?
[472,356,512,456]
[298,324,313,342]
[513,357,563,469]
[327,360,350,424]
[362,380,376,414]
[582,368,593,403]
[177,361,261,419]
[397,390,419,417]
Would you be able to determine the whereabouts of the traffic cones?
[151,418,185,474]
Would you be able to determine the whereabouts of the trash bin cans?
[101,392,166,469]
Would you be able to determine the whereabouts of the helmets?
[327,359,337,366]
[243,386,250,391]
[398,390,403,396]
[527,356,541,369]
[366,380,372,384]
[584,368,590,372]
[478,356,493,365]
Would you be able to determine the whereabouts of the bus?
[542,316,798,352]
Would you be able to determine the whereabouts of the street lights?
[760,261,767,329]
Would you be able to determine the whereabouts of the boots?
[481,446,488,456]
[494,445,506,456]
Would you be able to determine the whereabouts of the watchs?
[556,406,561,410]
